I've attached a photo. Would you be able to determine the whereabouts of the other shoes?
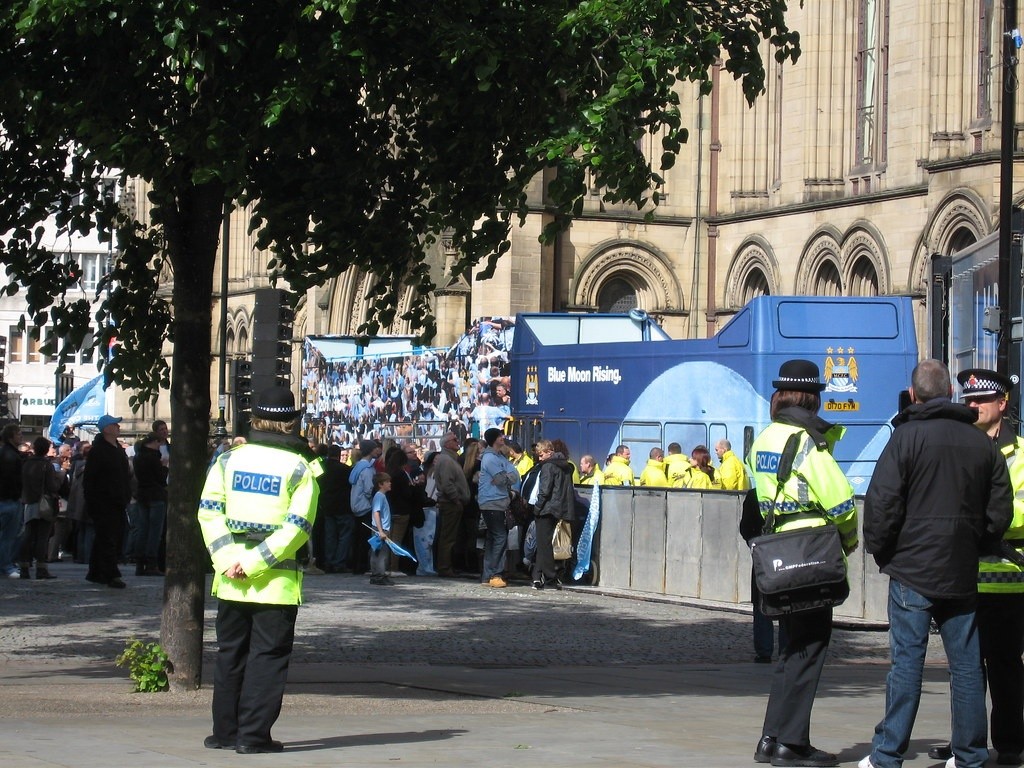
[135,558,165,576]
[436,565,506,587]
[36,563,57,579]
[8,572,20,578]
[858,755,874,768]
[20,562,30,579]
[85,566,126,588]
[532,580,544,590]
[754,656,771,663]
[945,751,957,768]
[324,562,343,573]
[370,575,393,585]
[351,563,370,575]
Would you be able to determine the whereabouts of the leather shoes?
[928,744,953,759]
[204,735,236,751]
[771,743,839,767]
[754,735,775,762]
[237,740,284,754]
[996,753,1021,765]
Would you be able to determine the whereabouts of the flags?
[367,532,415,560]
[572,473,599,580]
[48,374,106,445]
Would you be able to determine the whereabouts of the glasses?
[407,451,417,454]
[444,438,457,443]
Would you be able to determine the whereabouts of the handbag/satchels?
[748,525,850,620]
[38,497,55,523]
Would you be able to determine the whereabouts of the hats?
[98,415,122,432]
[252,386,301,422]
[484,428,504,446]
[772,360,826,392]
[359,440,378,456]
[957,369,1013,399]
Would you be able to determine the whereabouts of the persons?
[301,316,513,457]
[196,386,320,753]
[746,359,859,767]
[957,369,1024,768]
[858,362,1014,768]
[0,415,752,592]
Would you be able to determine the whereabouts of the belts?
[1005,539,1024,548]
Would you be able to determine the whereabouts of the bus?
[512,296,918,493]
[300,316,520,464]
